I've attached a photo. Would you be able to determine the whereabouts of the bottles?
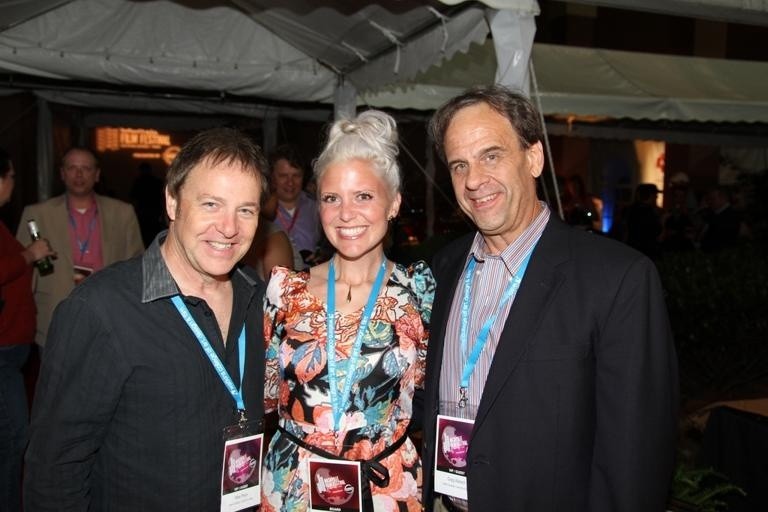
[26,218,55,278]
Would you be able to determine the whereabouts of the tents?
[344,42,768,150]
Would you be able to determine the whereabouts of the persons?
[1,144,146,510]
[23,84,674,511]
[651,176,767,404]
